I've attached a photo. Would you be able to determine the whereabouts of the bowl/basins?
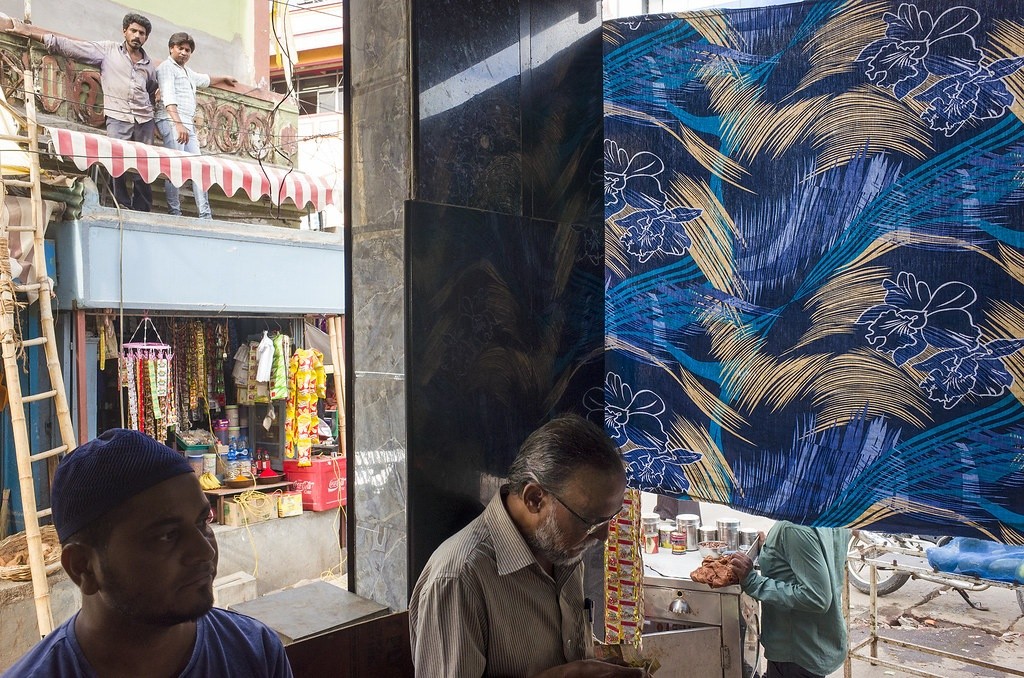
[698,541,729,560]
[254,473,285,485]
[223,477,254,488]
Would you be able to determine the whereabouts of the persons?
[407,415,659,678]
[154,32,238,219]
[731,519,848,678]
[1,429,296,678]
[5,13,159,212]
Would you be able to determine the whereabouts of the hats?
[51,428,195,543]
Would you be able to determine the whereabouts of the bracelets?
[39,34,43,43]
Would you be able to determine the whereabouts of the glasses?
[543,490,624,535]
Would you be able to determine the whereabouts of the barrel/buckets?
[641,513,757,553]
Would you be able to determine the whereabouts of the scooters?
[846,526,1024,616]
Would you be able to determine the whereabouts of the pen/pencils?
[584,597,594,623]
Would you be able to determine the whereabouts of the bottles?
[227,434,270,476]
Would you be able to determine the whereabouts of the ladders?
[2,68,81,637]
